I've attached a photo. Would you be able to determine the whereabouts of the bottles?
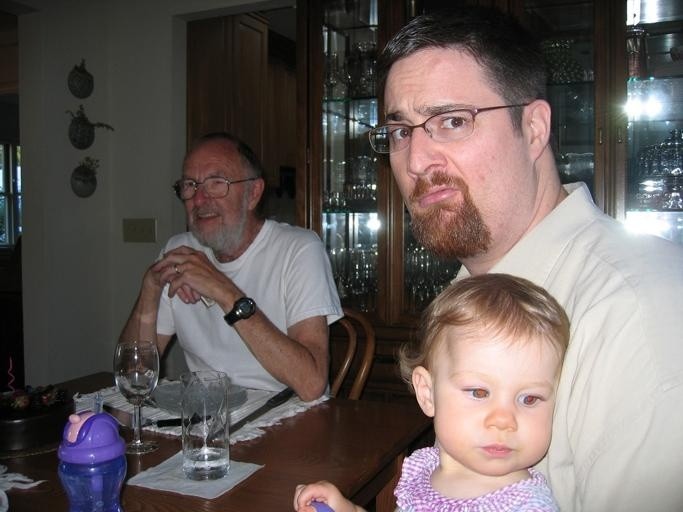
[626,26,647,78]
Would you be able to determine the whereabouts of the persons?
[290,268,569,510]
[111,125,347,401]
[367,1,681,511]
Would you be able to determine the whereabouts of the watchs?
[222,295,256,325]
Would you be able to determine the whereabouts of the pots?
[647,34,682,76]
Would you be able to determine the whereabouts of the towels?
[73,378,328,445]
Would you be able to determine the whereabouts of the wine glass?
[324,33,461,315]
[113,341,161,455]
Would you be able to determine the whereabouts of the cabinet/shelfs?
[186,0,682,402]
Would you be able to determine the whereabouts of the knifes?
[211,386,295,439]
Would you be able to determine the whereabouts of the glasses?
[173,176,254,201]
[368,103,528,154]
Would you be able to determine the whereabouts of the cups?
[54,410,128,512]
[177,370,230,481]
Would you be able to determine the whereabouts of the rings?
[172,262,179,273]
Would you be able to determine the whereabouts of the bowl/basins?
[0,386,70,449]
